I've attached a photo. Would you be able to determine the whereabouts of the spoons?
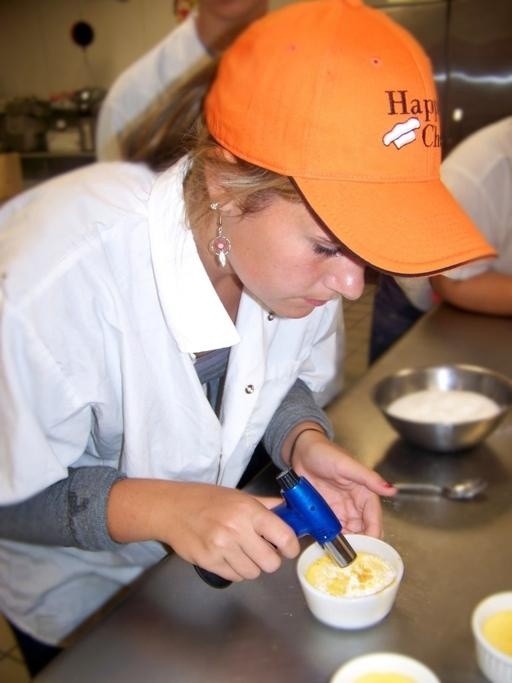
[389,476,490,501]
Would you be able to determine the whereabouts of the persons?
[94,0,273,163]
[0,0,499,681]
[365,108,509,366]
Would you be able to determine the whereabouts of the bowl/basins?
[468,590,512,683]
[368,361,512,454]
[295,532,407,631]
[328,651,442,683]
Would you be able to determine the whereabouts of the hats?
[204,0,500,279]
